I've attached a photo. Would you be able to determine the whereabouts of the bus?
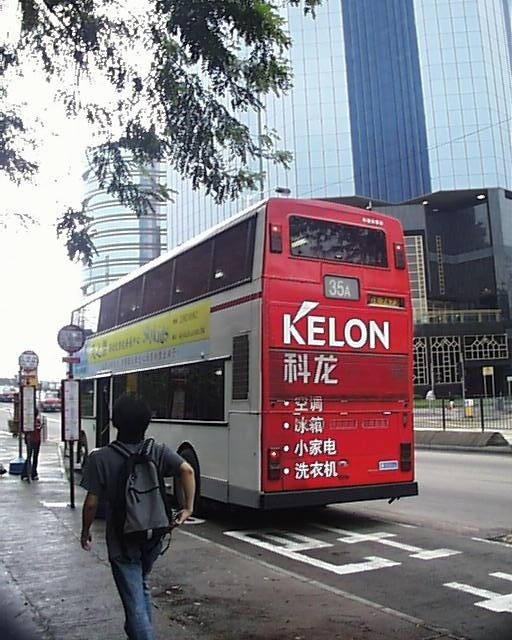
[68,196,417,523]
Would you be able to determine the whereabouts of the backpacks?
[108,438,173,540]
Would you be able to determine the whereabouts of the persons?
[79,393,196,640]
[22,410,42,481]
